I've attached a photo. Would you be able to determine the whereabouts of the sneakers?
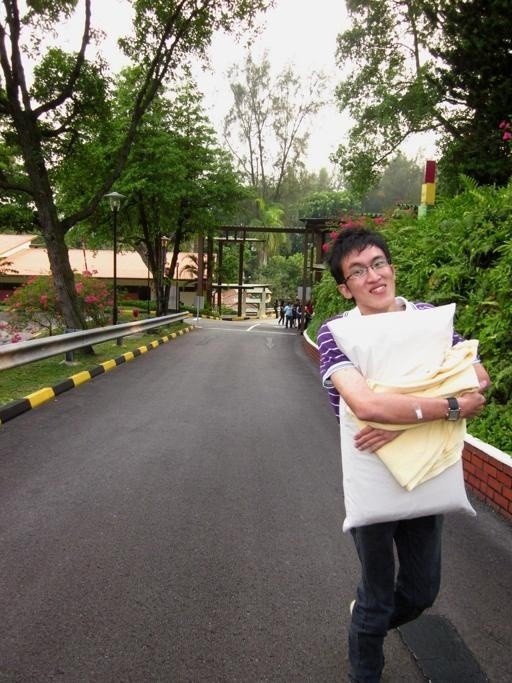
[350,600,357,616]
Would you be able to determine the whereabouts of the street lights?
[158,233,171,317]
[103,190,129,330]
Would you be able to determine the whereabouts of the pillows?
[325,300,475,533]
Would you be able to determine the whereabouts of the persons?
[274,298,312,328]
[316,226,490,683]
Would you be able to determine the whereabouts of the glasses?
[343,259,388,284]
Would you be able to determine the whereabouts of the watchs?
[445,398,462,421]
